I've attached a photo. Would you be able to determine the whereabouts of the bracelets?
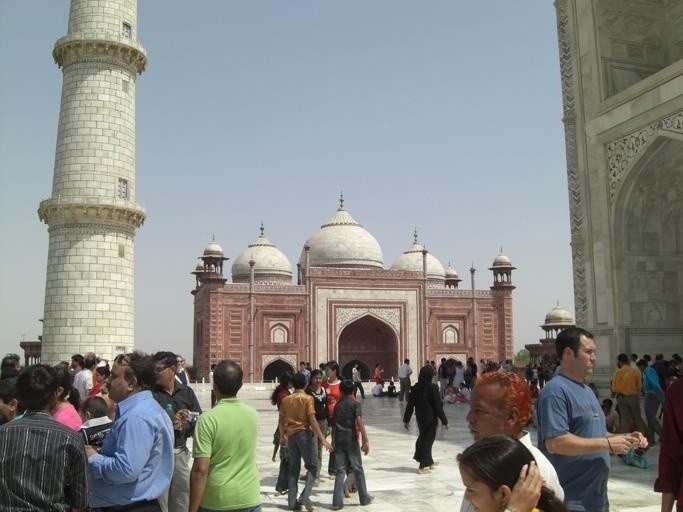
[606,438,612,453]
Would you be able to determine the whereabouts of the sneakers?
[430,463,439,468]
[418,469,430,474]
[275,474,375,511]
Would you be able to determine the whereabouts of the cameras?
[187,412,199,423]
[632,442,640,449]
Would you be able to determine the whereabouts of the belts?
[173,447,186,454]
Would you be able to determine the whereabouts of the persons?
[535,328,648,512]
[403,365,449,474]
[0,346,373,512]
[652,378,682,512]
[601,348,682,455]
[458,370,565,512]
[455,432,567,512]
[374,356,559,403]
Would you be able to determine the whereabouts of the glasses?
[155,366,170,371]
[114,354,132,365]
